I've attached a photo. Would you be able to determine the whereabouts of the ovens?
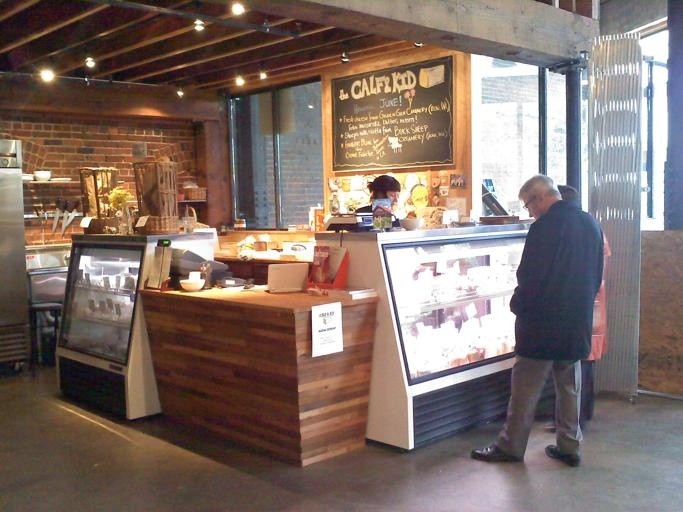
[0,140,31,375]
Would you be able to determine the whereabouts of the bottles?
[288,225,297,232]
[330,189,340,214]
[234,219,246,230]
[220,226,226,236]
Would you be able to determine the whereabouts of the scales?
[325,213,374,233]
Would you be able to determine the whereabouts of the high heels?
[542,409,592,433]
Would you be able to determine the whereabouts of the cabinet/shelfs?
[214,229,315,284]
[313,225,558,451]
[53,227,216,422]
[24,242,71,379]
[22,180,84,219]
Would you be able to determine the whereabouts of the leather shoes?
[470,443,523,463]
[545,444,580,465]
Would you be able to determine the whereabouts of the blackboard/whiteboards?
[331,55,456,173]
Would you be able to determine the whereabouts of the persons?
[541,185,613,436]
[354,174,401,228]
[466,172,602,466]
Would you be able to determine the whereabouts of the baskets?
[180,206,209,231]
[182,186,207,200]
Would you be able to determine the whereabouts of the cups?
[176,217,192,233]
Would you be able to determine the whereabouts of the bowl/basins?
[400,218,424,231]
[34,171,51,182]
[179,280,206,292]
[22,174,34,181]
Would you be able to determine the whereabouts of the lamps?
[341,41,351,63]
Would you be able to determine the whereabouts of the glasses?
[523,195,536,211]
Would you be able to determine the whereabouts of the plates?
[51,177,72,181]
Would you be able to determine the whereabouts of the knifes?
[36,197,80,237]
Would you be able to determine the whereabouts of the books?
[326,247,346,282]
[312,245,329,282]
[327,287,378,295]
[328,292,377,300]
